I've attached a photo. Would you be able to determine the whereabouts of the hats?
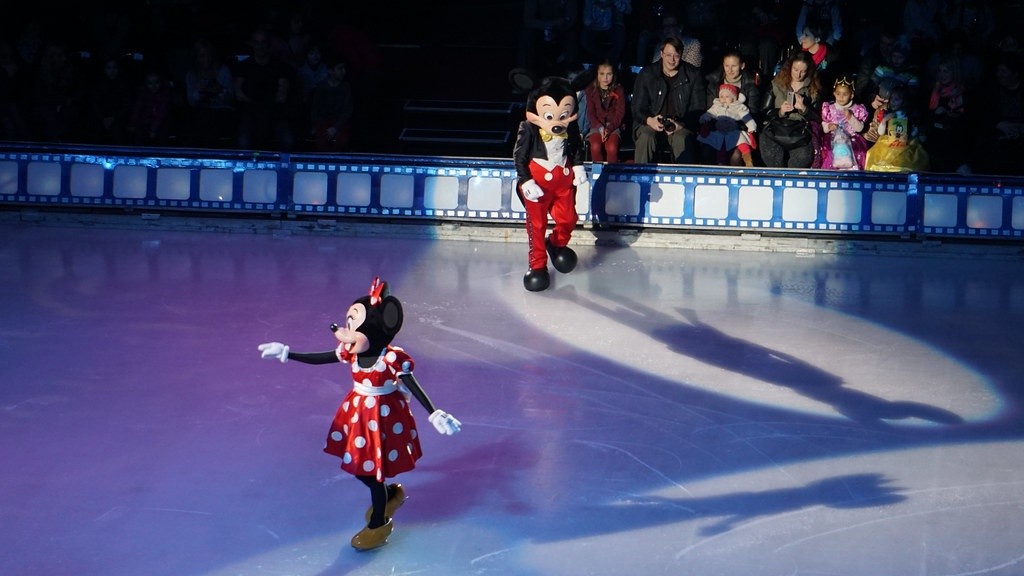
[892,34,911,58]
[719,85,739,102]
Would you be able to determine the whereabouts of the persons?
[522,1,1023,176]
[258,275,461,550]
[510,67,597,293]
[0,0,371,151]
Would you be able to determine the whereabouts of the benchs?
[581,130,717,162]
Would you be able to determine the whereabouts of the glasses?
[663,52,680,59]
[662,23,680,28]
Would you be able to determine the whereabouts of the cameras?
[659,114,680,131]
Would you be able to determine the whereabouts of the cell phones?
[787,92,794,106]
